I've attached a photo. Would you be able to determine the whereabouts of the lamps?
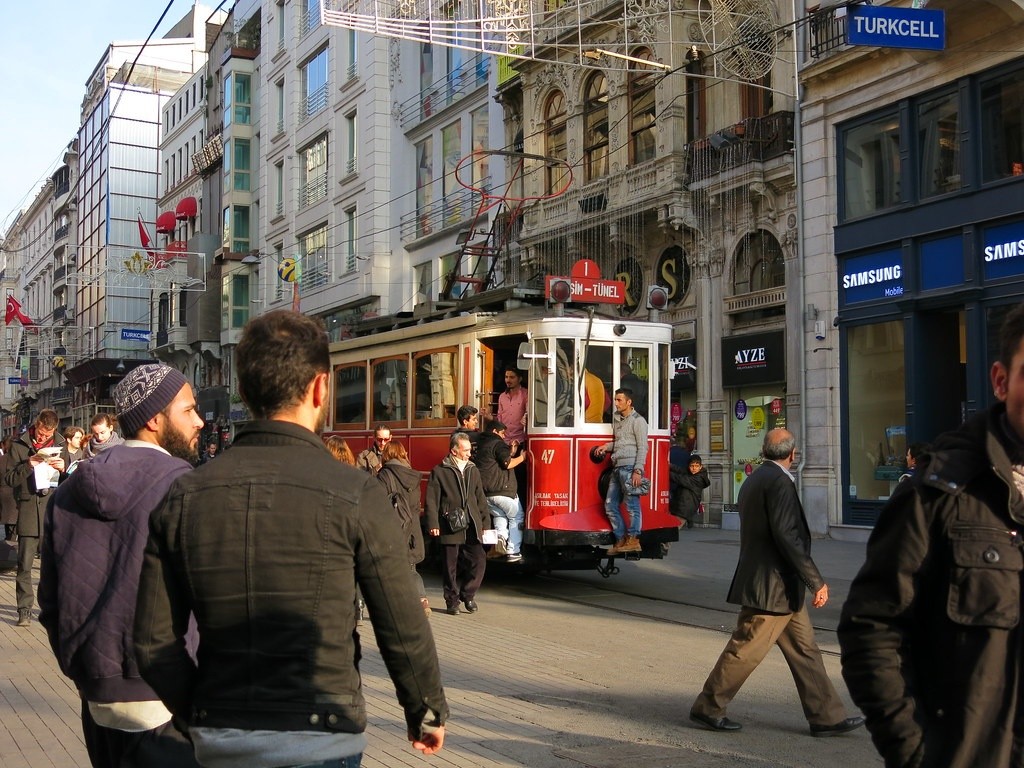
[242,253,260,264]
[115,359,126,373]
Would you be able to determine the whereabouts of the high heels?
[354,596,364,619]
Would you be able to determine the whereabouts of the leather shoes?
[809,717,865,737]
[447,604,460,614]
[689,708,742,731]
[459,594,478,613]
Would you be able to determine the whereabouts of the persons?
[200,442,231,463]
[457,404,527,562]
[38,364,204,768]
[134,311,449,768]
[355,424,392,476]
[424,433,492,615]
[0,409,124,626]
[621,362,648,419]
[350,362,431,422]
[480,367,528,507]
[690,429,866,736]
[378,440,433,616]
[594,389,649,555]
[838,303,1024,768]
[562,360,611,423]
[661,435,710,555]
[326,435,363,622]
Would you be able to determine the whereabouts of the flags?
[5,298,34,330]
[138,217,154,256]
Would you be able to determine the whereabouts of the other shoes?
[487,543,501,558]
[419,597,432,618]
[17,609,31,626]
[33,551,39,559]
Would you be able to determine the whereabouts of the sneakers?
[494,535,507,555]
[607,538,625,555]
[507,553,522,562]
[618,536,642,552]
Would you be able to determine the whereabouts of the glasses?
[375,435,390,443]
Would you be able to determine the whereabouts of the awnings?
[156,211,176,234]
[175,197,197,221]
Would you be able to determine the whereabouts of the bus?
[319,149,685,577]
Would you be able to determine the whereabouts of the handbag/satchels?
[384,468,412,530]
[444,508,467,533]
[696,502,705,516]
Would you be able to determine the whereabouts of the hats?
[114,363,188,438]
